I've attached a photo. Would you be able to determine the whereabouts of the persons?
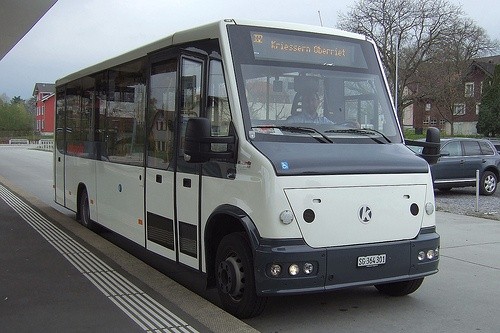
[285,90,335,124]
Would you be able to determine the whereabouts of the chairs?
[286,89,320,122]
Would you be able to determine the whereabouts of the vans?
[407,138,500,196]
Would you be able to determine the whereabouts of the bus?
[51,18,440,318]
